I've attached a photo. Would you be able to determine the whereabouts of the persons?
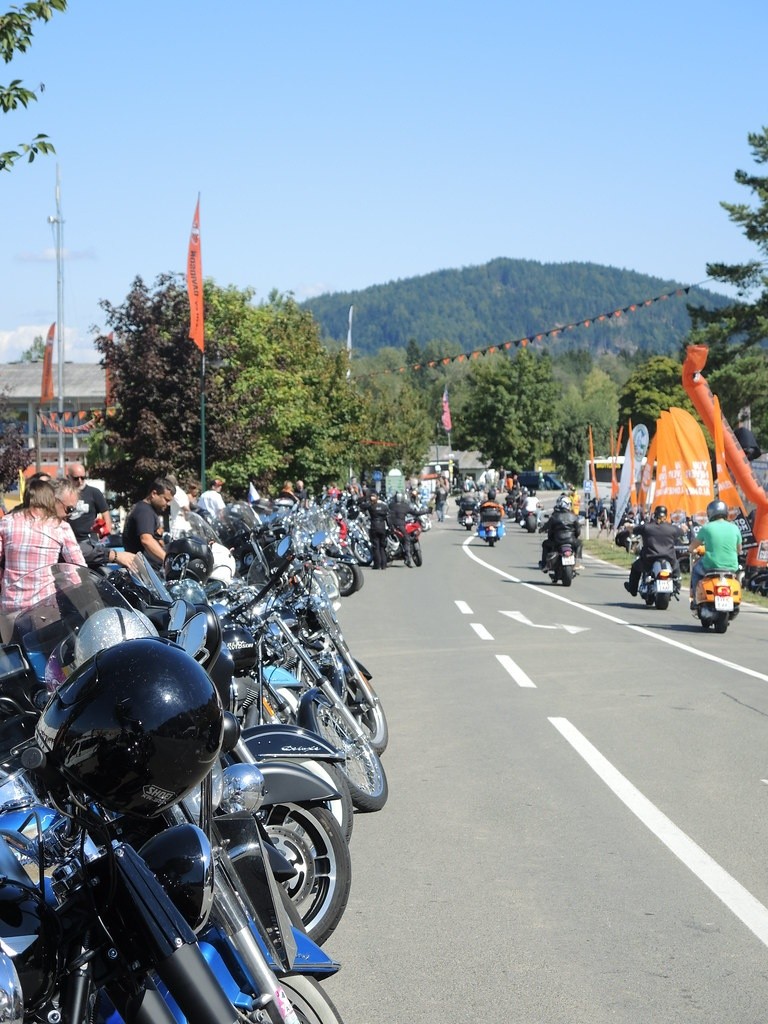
[456,488,479,518]
[499,465,505,494]
[506,475,543,522]
[464,476,477,492]
[33,473,139,573]
[281,480,307,503]
[688,500,742,611]
[540,496,583,573]
[430,476,449,522]
[389,493,432,568]
[624,505,687,597]
[122,477,176,564]
[358,493,389,570]
[322,477,367,496]
[0,478,88,643]
[480,490,500,510]
[164,475,226,532]
[64,463,112,544]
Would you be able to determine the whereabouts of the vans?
[506,471,570,491]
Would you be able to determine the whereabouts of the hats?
[210,479,224,486]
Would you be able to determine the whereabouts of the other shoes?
[624,581,638,596]
[538,561,548,574]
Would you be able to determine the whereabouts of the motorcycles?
[629,533,680,610]
[455,498,480,532]
[502,487,544,533]
[615,524,768,596]
[474,502,506,548]
[0,489,440,1024]
[683,545,749,636]
[536,518,580,587]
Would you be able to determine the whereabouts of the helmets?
[395,492,404,502]
[348,505,359,520]
[512,485,518,490]
[556,495,572,510]
[476,486,481,491]
[706,500,728,520]
[74,607,160,670]
[488,491,496,499]
[530,489,536,497]
[33,639,225,832]
[521,486,528,494]
[654,506,667,518]
[162,535,215,583]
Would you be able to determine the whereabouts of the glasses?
[54,497,74,514]
[69,473,87,481]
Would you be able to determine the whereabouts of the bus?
[583,456,657,516]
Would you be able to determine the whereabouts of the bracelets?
[112,550,117,563]
